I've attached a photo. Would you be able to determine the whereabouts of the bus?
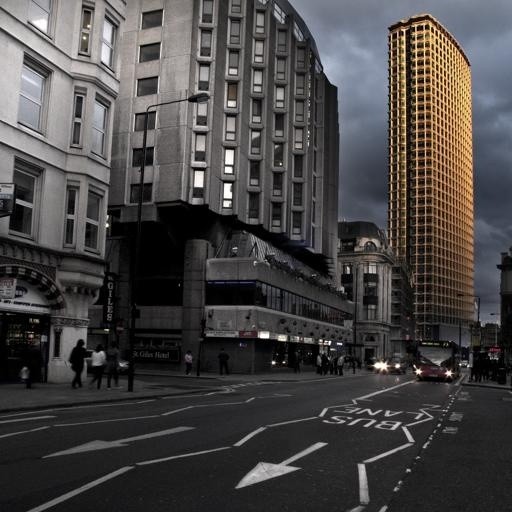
[416,340,460,382]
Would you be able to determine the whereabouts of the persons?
[217,347,232,376]
[106,339,123,391]
[293,350,302,373]
[88,341,108,391]
[314,351,374,376]
[184,348,194,376]
[68,338,92,389]
[465,351,512,385]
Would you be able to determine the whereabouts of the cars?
[460,361,467,368]
[376,355,408,376]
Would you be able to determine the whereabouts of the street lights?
[457,293,481,328]
[127,92,210,391]
